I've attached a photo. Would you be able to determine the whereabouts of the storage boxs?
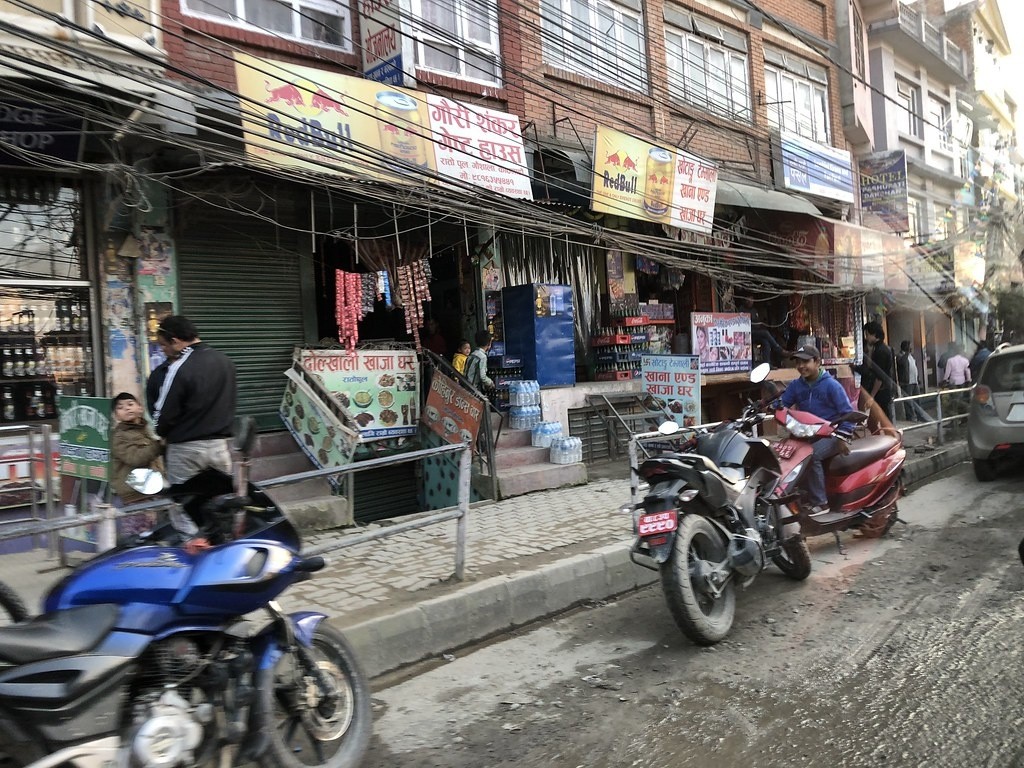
[486,355,523,369]
[490,398,510,411]
[593,304,673,380]
[482,375,523,391]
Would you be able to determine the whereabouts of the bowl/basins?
[354,391,372,406]
[377,391,394,408]
[379,375,395,388]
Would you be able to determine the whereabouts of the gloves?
[832,427,853,442]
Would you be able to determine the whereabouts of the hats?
[790,344,821,360]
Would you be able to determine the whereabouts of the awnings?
[748,207,908,291]
[533,148,824,215]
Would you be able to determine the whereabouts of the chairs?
[1012,362,1024,388]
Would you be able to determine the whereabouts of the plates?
[282,379,334,465]
[331,391,350,410]
[669,399,682,413]
[644,396,667,412]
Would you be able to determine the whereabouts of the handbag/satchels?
[935,381,951,408]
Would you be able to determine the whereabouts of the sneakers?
[802,503,830,517]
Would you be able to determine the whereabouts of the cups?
[401,405,408,425]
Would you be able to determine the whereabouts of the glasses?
[155,323,176,337]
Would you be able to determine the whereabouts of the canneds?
[374,91,428,175]
[643,147,673,218]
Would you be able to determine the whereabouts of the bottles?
[797,335,816,350]
[594,309,649,372]
[0,302,89,334]
[0,343,93,378]
[2,387,14,422]
[487,369,582,465]
[27,383,90,419]
[410,396,416,425]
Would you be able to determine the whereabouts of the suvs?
[966,341,1024,480]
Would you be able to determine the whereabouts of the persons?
[451,339,471,385]
[696,326,709,362]
[938,341,991,428]
[465,329,496,455]
[420,316,447,352]
[109,392,165,540]
[850,321,898,438]
[760,345,857,516]
[145,315,237,541]
[895,340,928,422]
[735,295,794,370]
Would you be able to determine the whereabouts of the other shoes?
[943,421,955,429]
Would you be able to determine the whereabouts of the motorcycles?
[1,416,372,768]
[629,361,813,646]
[763,379,907,538]
